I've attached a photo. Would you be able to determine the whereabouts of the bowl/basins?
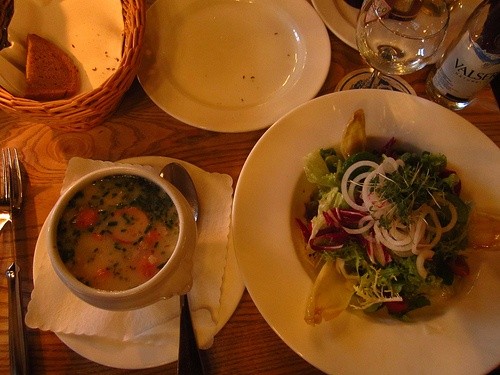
[46,167,197,313]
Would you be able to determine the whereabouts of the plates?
[311,0,490,64]
[134,0,331,134]
[229,89,499,375]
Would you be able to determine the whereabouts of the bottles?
[425,0,500,112]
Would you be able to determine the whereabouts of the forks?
[0,148,28,375]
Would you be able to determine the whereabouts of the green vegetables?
[57,176,177,290]
[298,144,471,326]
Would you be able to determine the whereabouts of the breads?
[21,32,81,98]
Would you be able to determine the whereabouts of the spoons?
[158,162,206,375]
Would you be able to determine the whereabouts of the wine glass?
[351,0,450,92]
